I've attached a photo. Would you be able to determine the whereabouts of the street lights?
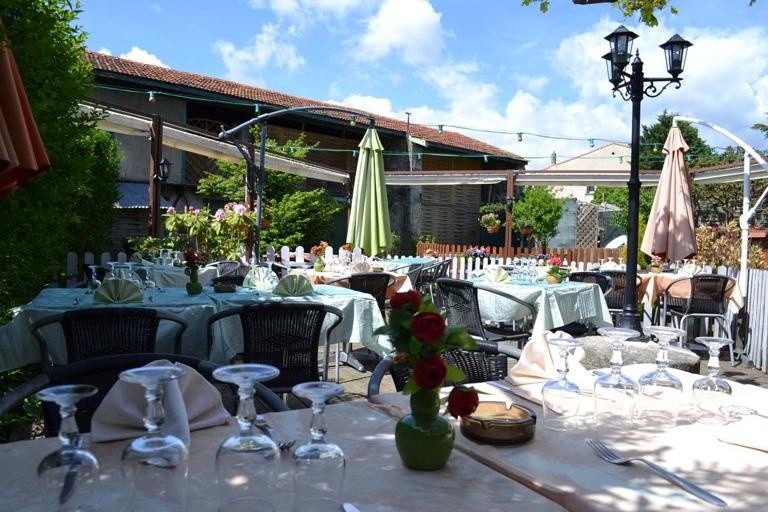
[148,156,175,242]
[503,195,514,259]
[598,24,695,343]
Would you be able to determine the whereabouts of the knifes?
[56,430,85,502]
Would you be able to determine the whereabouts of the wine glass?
[33,383,101,512]
[155,247,179,268]
[212,365,281,512]
[115,366,192,512]
[539,325,733,432]
[597,256,708,275]
[86,260,157,302]
[244,258,310,293]
[510,257,539,284]
[287,382,348,511]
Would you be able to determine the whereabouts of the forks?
[243,413,297,451]
[585,435,729,508]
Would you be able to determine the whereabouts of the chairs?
[1,241,767,511]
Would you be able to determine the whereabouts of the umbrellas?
[219,106,393,265]
[640,117,767,351]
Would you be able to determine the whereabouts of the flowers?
[150,201,254,238]
